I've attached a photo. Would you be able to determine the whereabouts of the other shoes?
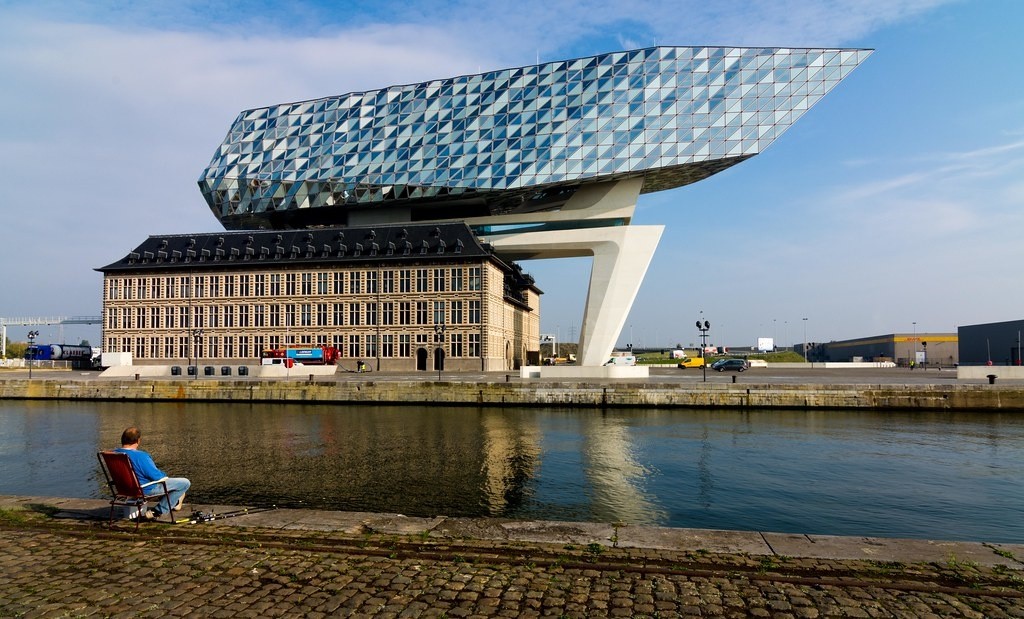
[173,492,185,511]
[145,510,168,520]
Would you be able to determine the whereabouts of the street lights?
[803,318,808,365]
[696,320,710,382]
[195,329,205,380]
[434,324,447,382]
[27,330,38,379]
[912,323,916,363]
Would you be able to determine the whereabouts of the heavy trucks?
[24,344,92,370]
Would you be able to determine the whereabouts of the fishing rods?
[176,501,334,525]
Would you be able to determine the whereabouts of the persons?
[905,360,915,371]
[113,428,191,518]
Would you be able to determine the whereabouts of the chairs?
[97,452,175,533]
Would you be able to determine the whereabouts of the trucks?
[705,346,728,357]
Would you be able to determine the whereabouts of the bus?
[260,344,342,366]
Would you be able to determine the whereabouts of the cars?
[636,356,646,362]
[711,358,748,372]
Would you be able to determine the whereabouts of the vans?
[604,356,637,366]
[680,358,704,369]
[669,350,687,359]
